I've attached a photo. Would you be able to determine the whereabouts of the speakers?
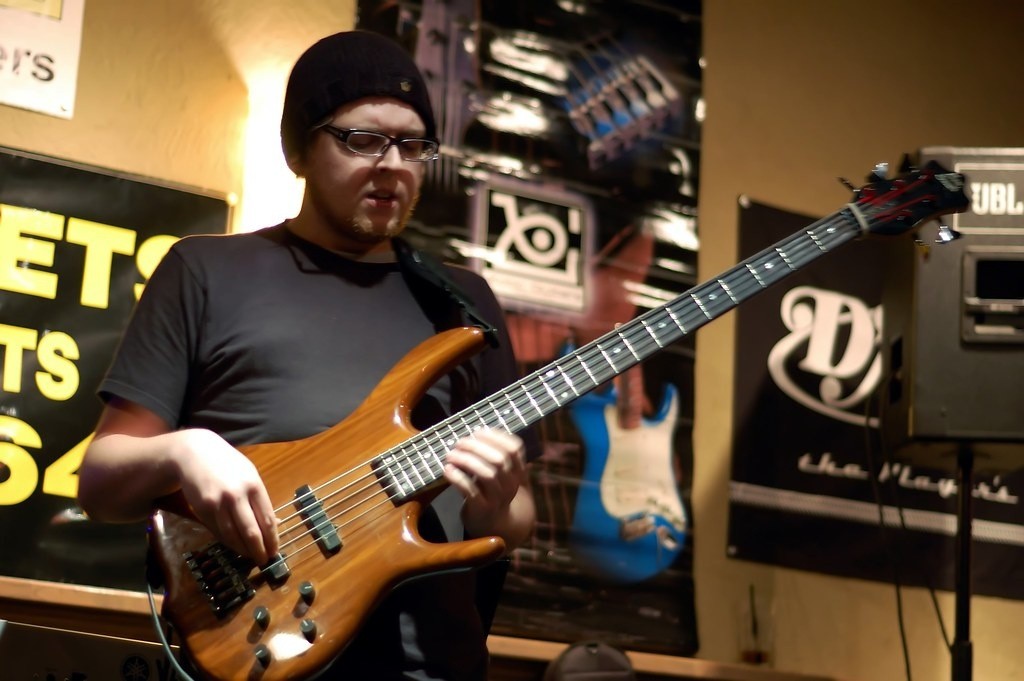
[903,147,1024,444]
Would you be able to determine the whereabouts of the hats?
[283,31,436,151]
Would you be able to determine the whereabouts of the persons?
[77,32,545,681]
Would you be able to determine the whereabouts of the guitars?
[566,138,692,586]
[145,145,976,681]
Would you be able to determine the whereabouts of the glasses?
[319,122,440,162]
